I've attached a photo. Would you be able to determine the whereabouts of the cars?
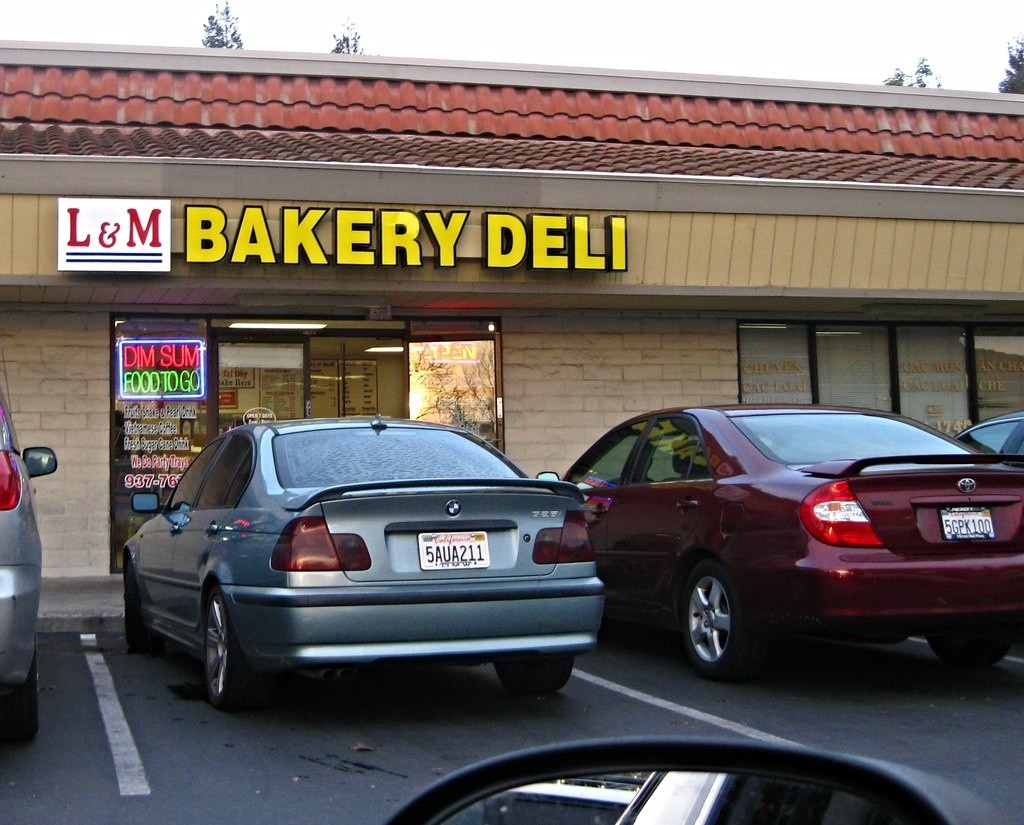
[122,419,608,712]
[537,402,1024,681]
[1,395,59,743]
